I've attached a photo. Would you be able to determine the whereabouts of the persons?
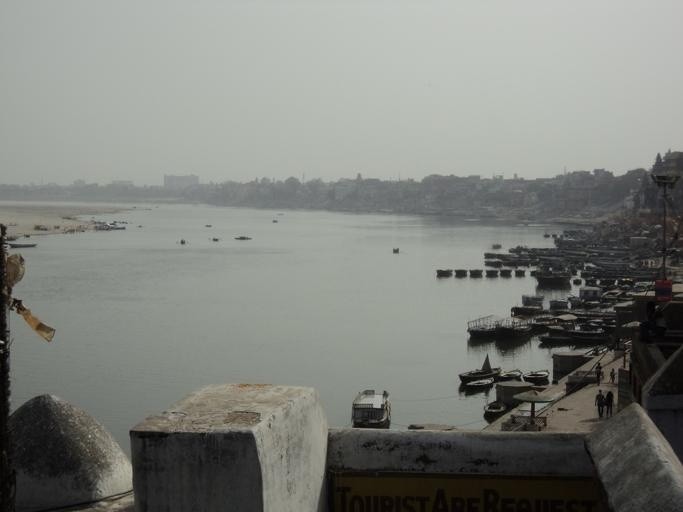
[595,363,615,386]
[595,390,605,416]
[604,391,613,417]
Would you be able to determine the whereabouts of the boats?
[8,242,37,249]
[482,228,632,267]
[516,270,656,308]
[348,388,393,429]
[435,268,511,279]
[454,351,550,418]
[464,309,618,346]
[95,222,127,232]
[233,236,252,241]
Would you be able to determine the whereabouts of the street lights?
[650,172,680,280]
